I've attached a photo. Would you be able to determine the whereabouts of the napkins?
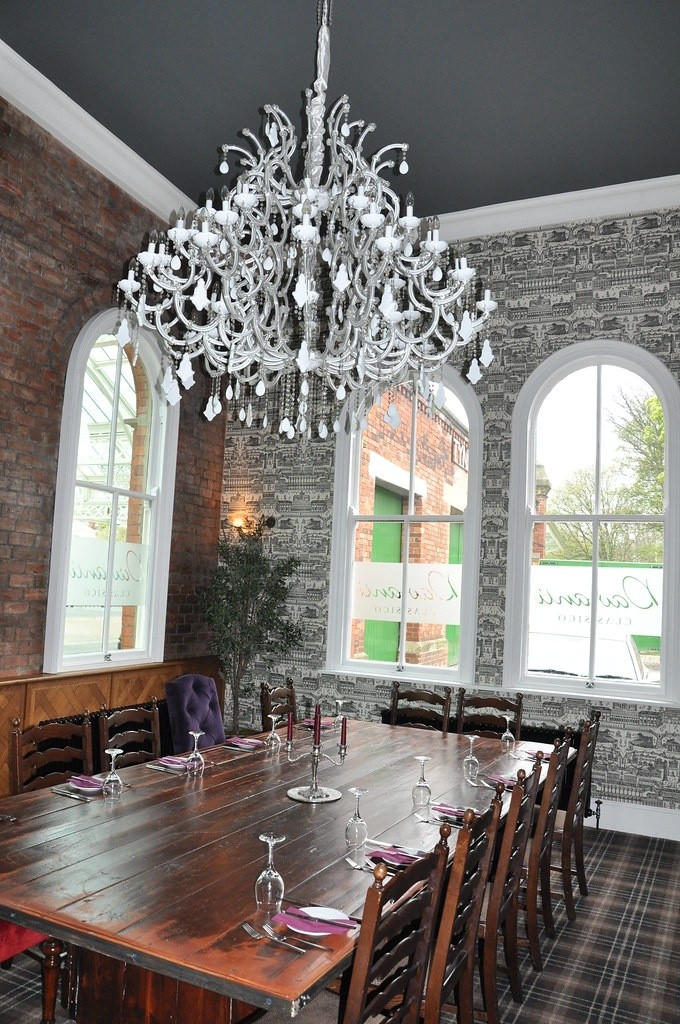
[67,774,117,788]
[488,773,516,787]
[365,847,418,864]
[525,750,550,760]
[225,736,265,747]
[271,907,356,935]
[303,719,333,726]
[432,802,482,818]
[158,755,202,769]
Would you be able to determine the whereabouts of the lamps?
[114,0,498,440]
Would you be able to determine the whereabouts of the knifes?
[364,845,424,860]
[281,909,357,931]
[52,786,92,802]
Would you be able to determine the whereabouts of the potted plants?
[197,515,303,739]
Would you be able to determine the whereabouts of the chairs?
[10,682,602,1024]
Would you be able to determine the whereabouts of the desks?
[0,715,578,1024]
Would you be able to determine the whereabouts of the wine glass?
[501,711,515,753]
[254,831,285,913]
[411,754,432,808]
[102,749,124,804]
[187,730,206,779]
[345,787,369,847]
[265,713,282,756]
[463,735,479,779]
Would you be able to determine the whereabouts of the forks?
[345,856,404,878]
[242,922,333,955]
[414,813,465,830]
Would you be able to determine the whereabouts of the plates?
[285,906,349,935]
[70,776,107,793]
[231,738,262,749]
[380,848,425,867]
[158,756,192,770]
